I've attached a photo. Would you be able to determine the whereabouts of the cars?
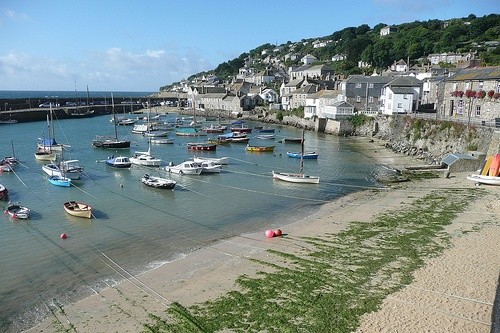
[39,102,55,108]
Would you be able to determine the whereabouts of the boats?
[4,199,31,219]
[286,151,318,160]
[141,174,177,190]
[164,160,203,175]
[4,140,18,162]
[193,155,229,165]
[42,159,85,180]
[255,134,275,139]
[199,161,222,173]
[106,156,132,168]
[0,183,7,200]
[467,173,500,186]
[63,200,92,219]
[0,159,13,172]
[255,128,275,133]
[246,144,276,152]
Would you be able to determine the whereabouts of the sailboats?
[48,145,72,187]
[128,105,164,166]
[70,84,95,118]
[109,91,252,150]
[37,102,64,152]
[91,91,131,148]
[34,114,57,162]
[272,126,320,184]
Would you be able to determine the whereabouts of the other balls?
[265,230,274,237]
[274,229,282,236]
